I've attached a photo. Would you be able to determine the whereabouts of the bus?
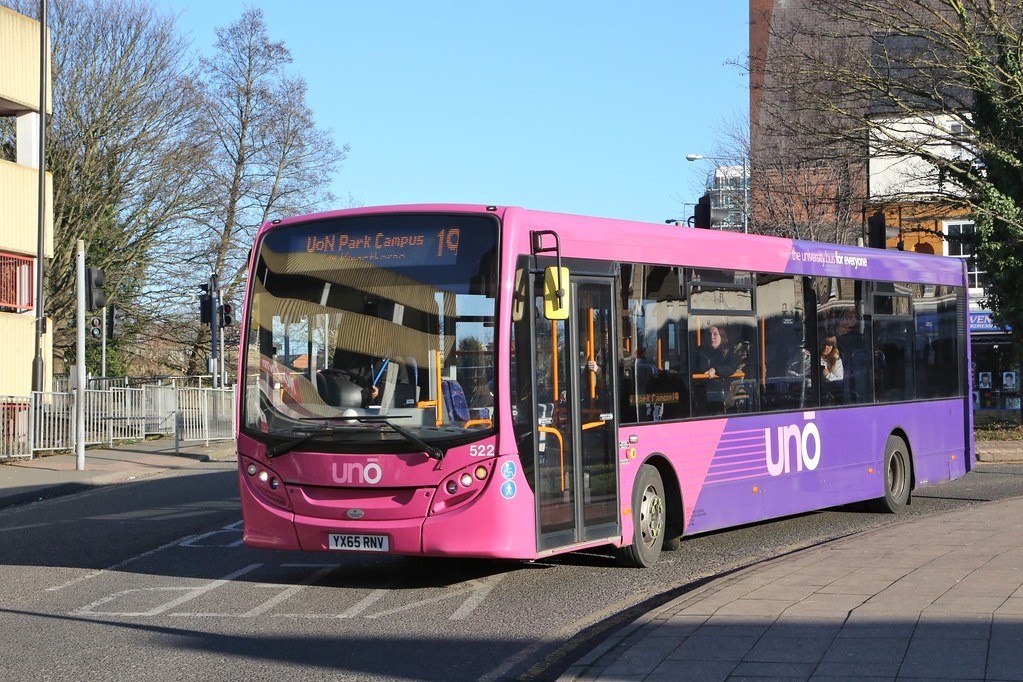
[235,201,977,571]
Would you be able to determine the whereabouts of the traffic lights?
[221,303,234,326]
[90,317,101,339]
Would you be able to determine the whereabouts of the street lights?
[685,152,751,234]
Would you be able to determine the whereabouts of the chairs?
[315,331,891,432]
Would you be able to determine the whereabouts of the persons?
[355,323,409,406]
[1004,373,1015,389]
[979,373,990,388]
[784,332,845,390]
[560,323,633,409]
[694,322,738,377]
